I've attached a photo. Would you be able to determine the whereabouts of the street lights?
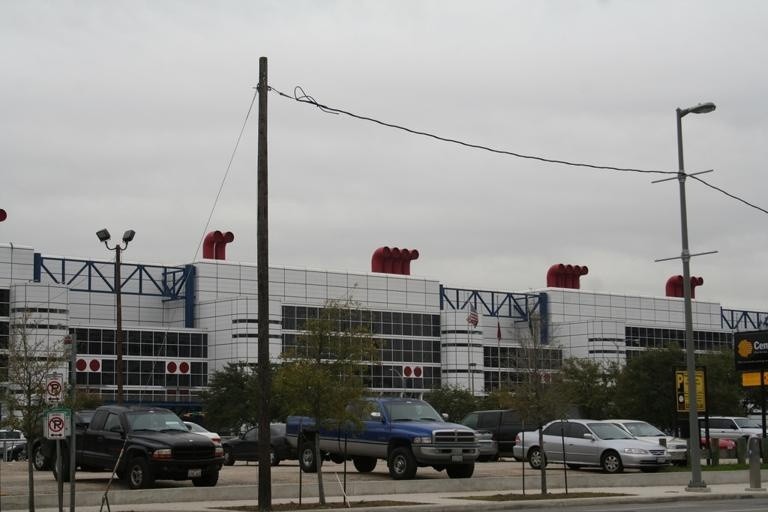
[674,100,719,495]
[467,362,477,395]
[93,226,138,404]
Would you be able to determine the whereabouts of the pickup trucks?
[279,395,483,481]
[50,403,227,491]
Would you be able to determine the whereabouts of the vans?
[459,403,550,459]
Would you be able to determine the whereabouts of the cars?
[1,417,297,471]
[511,413,767,476]
[466,427,497,458]
